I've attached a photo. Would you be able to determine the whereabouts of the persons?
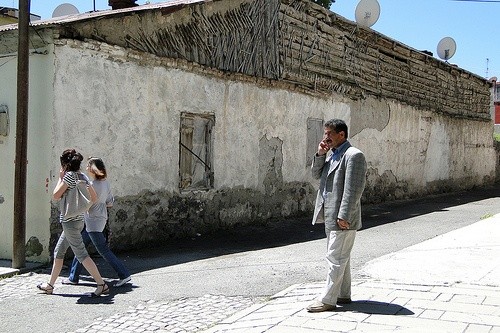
[61,157,132,288]
[36,148,109,298]
[306,118,368,313]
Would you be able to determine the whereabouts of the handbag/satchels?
[60,172,93,219]
[103,225,112,249]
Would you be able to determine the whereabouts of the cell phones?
[62,164,68,172]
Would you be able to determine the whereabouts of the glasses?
[87,156,92,160]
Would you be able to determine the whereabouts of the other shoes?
[337,298,351,304]
[59,278,77,284]
[306,301,336,311]
[113,276,131,286]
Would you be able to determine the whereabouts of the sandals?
[36,281,54,294]
[88,282,109,297]
